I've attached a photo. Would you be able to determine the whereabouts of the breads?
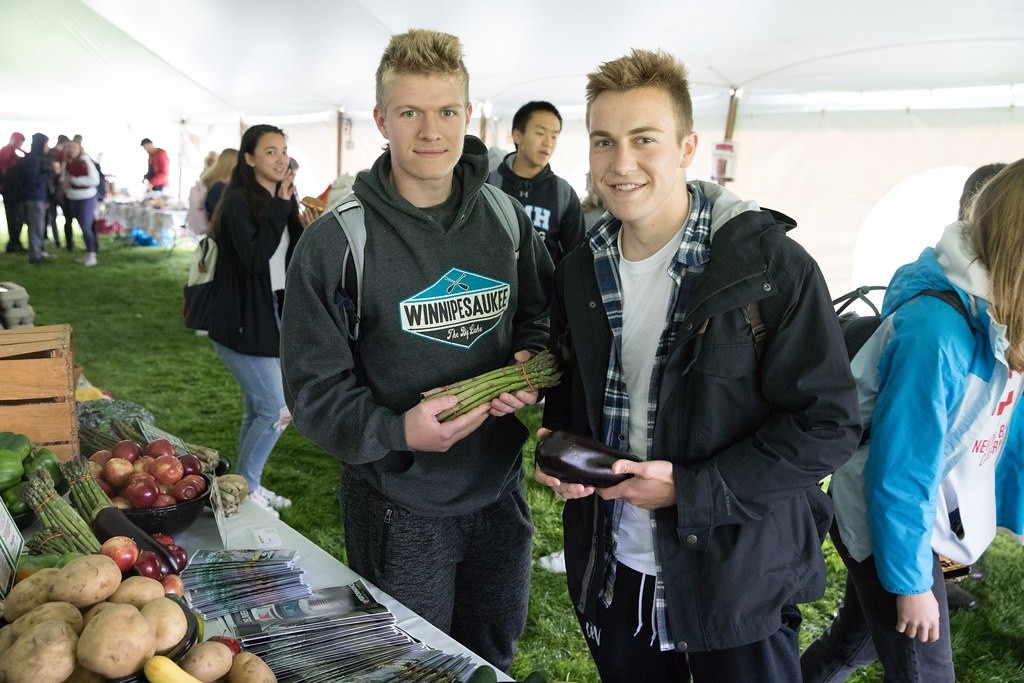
[302,196,326,212]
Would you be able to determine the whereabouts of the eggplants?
[536,430,644,488]
[101,592,200,683]
[93,507,180,575]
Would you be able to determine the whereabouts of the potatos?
[0,554,187,683]
[178,640,279,683]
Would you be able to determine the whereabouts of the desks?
[107,200,201,258]
[173,496,517,682]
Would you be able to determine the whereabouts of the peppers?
[0,431,64,516]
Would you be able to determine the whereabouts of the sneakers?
[247,491,279,519]
[259,487,292,511]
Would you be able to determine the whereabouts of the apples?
[205,629,241,657]
[100,532,188,599]
[84,438,206,509]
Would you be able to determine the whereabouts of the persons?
[280,27,552,673]
[801,158,1024,683]
[485,101,610,572]
[195,125,371,519]
[0,133,100,266]
[533,49,864,683]
[141,138,168,193]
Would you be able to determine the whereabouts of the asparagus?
[421,347,563,422]
[183,441,249,518]
[77,415,149,459]
[23,453,116,555]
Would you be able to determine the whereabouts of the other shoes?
[85,252,97,266]
[539,548,566,574]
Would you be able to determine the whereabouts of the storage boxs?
[0,322,80,465]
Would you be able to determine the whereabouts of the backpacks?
[182,181,211,234]
[828,286,977,365]
[183,237,218,330]
